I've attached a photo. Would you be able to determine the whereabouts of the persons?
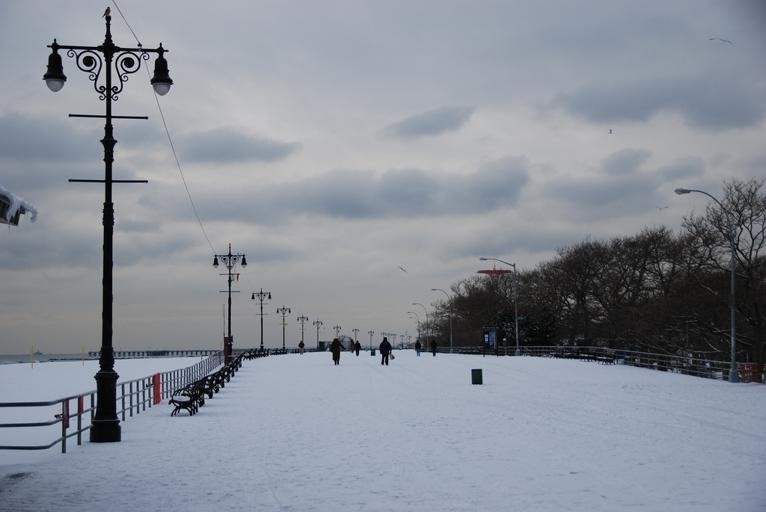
[415,340,421,356]
[299,341,304,355]
[380,337,392,365]
[331,339,344,365]
[354,341,361,356]
[351,339,354,353]
[431,340,437,356]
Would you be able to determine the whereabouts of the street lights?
[312,301,429,356]
[296,313,309,343]
[251,287,272,352]
[479,256,523,357]
[39,3,174,446]
[672,187,739,383]
[432,288,454,353]
[211,242,248,365]
[276,304,291,351]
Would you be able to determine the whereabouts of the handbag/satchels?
[390,353,394,360]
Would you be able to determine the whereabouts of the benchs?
[167,350,245,416]
[550,348,617,365]
